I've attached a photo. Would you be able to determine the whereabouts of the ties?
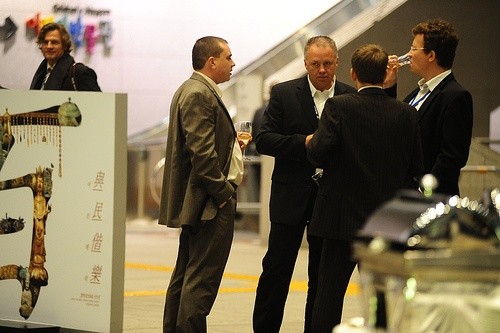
[41,67,54,90]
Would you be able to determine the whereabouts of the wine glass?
[236,120,252,160]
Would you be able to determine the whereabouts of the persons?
[28,23,103,92]
[252,35,359,333]
[157,38,253,333]
[306,19,474,333]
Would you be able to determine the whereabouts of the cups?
[387,52,411,70]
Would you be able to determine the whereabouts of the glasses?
[410,47,427,53]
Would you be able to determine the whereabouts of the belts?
[229,181,237,191]
